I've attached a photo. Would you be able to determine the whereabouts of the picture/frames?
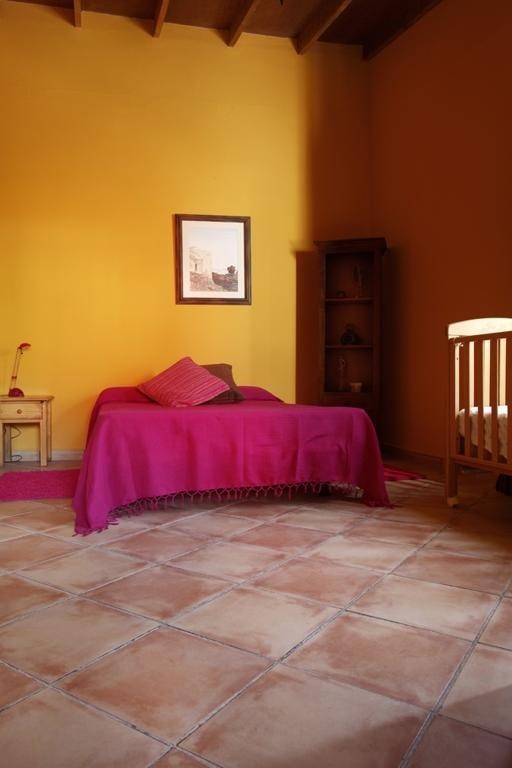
[175,213,252,305]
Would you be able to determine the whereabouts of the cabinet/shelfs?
[312,237,387,455]
[0,395,55,467]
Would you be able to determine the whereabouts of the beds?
[444,316,512,508]
[70,399,396,537]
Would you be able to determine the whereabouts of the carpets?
[0,469,80,502]
[383,462,422,482]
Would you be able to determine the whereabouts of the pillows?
[136,356,231,408]
[197,364,246,403]
[86,385,283,443]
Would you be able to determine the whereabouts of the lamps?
[7,342,32,397]
